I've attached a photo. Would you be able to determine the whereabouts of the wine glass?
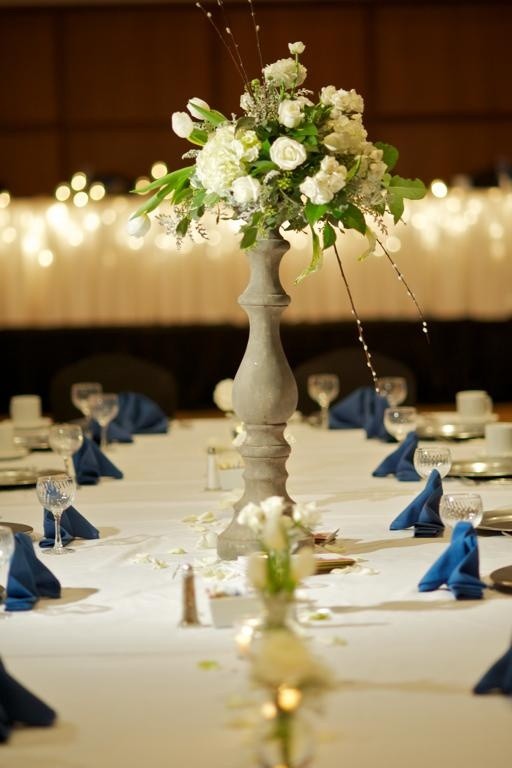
[1,525,15,619]
[307,369,340,438]
[49,426,82,489]
[73,382,121,459]
[36,476,75,556]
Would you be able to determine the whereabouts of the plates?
[476,507,511,532]
[445,457,512,478]
[1,464,67,490]
[1,519,35,537]
[1,392,55,462]
[417,408,501,439]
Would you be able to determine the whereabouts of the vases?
[216,229,303,568]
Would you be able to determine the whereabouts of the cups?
[456,391,491,419]
[414,448,448,482]
[383,409,416,446]
[486,423,512,451]
[373,376,407,407]
[440,493,481,531]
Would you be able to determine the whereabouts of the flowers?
[128,1,430,395]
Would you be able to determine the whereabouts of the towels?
[326,380,397,445]
[108,387,172,446]
